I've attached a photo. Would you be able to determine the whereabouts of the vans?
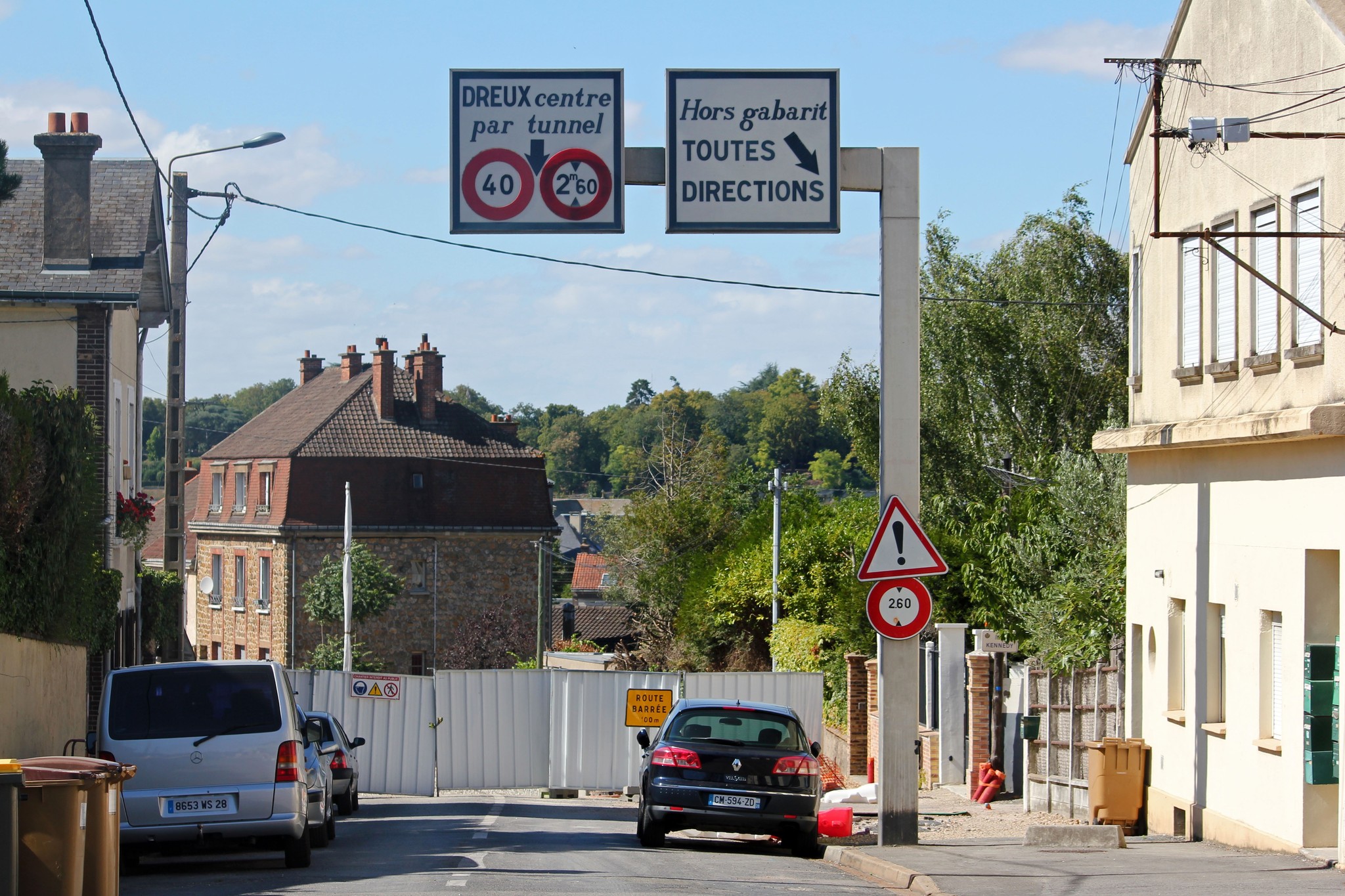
[84,660,324,868]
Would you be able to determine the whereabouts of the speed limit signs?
[453,67,626,236]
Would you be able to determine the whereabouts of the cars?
[306,711,365,815]
[296,704,339,848]
[637,699,824,856]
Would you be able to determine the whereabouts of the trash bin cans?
[1086,737,1152,835]
[0,756,138,896]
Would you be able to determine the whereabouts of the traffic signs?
[667,66,845,237]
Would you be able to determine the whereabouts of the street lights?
[168,134,285,251]
